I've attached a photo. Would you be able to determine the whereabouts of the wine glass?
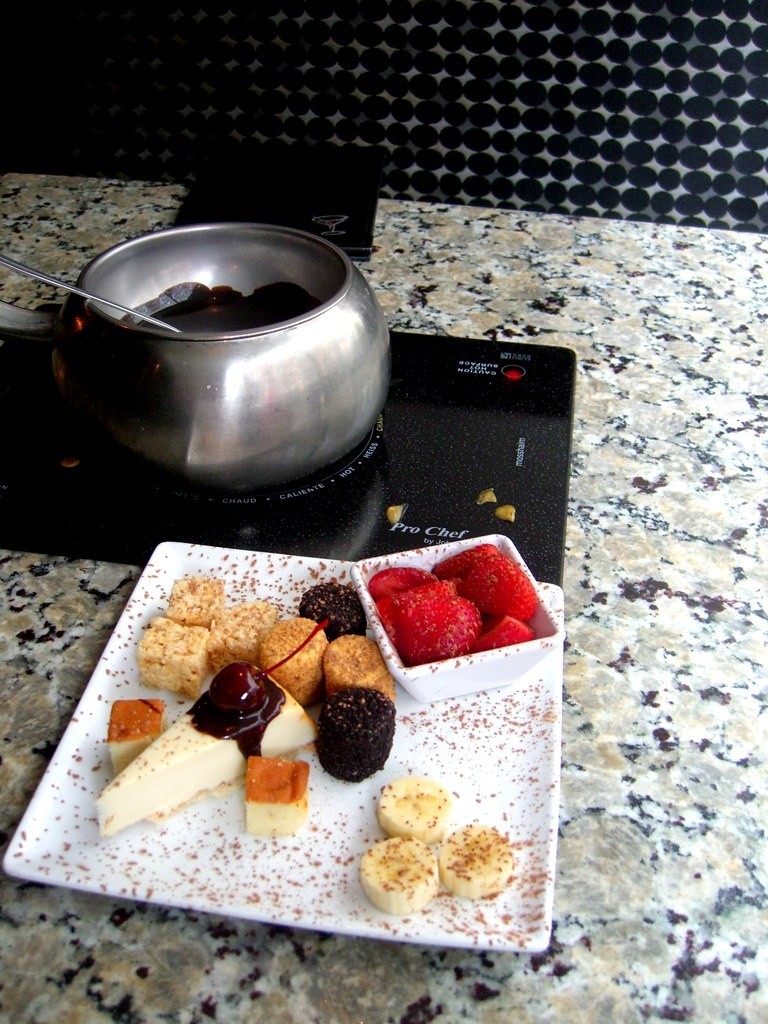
[312,215,349,236]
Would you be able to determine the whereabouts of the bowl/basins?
[351,534,566,702]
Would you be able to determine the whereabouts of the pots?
[53,223,391,490]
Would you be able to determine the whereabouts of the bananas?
[360,777,513,912]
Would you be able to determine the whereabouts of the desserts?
[95,665,318,833]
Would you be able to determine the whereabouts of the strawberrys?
[370,542,537,668]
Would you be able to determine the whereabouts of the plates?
[3,542,564,951]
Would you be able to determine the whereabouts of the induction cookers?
[0,303,575,584]
